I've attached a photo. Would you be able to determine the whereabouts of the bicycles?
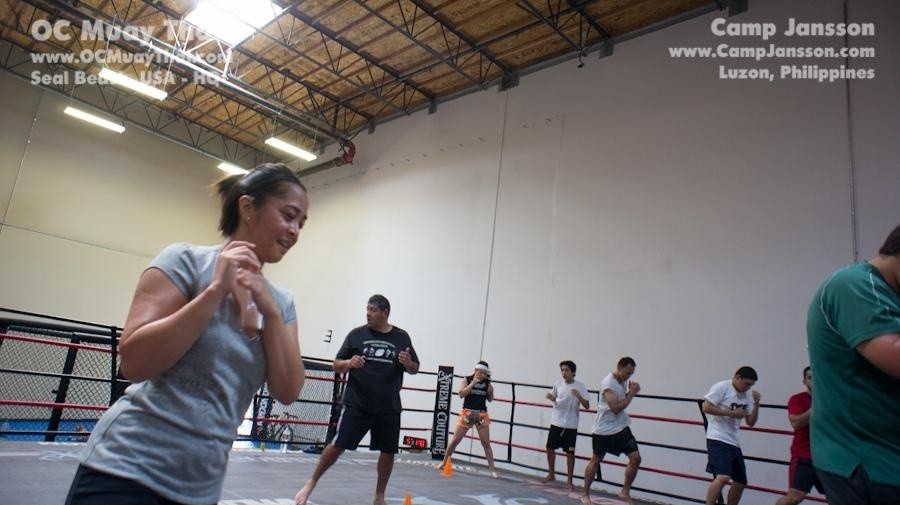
[259,411,299,443]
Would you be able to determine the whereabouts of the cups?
[260,443,265,451]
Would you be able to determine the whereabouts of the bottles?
[0,422,9,448]
[280,427,290,453]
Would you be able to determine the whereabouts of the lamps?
[216,162,247,175]
[98,14,168,101]
[265,109,317,162]
[63,85,126,134]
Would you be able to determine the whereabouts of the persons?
[701,367,762,505]
[541,361,589,497]
[295,294,419,504]
[63,163,309,504]
[436,362,500,479]
[775,367,829,504]
[807,223,900,504]
[580,357,642,504]
